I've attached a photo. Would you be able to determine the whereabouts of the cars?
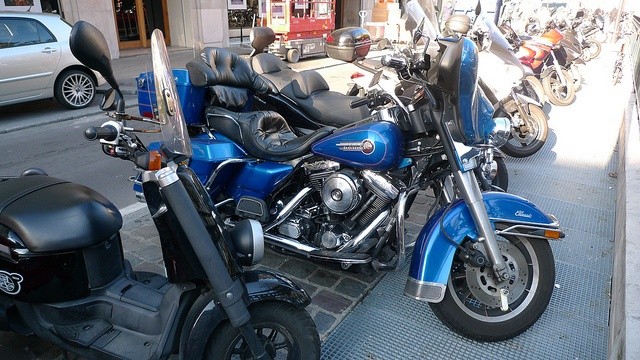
[0,1,109,110]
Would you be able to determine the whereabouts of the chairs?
[185,46,276,161]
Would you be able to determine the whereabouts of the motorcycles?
[107,0,566,346]
[519,0,592,63]
[322,1,551,164]
[0,20,322,360]
[578,6,606,60]
[492,0,575,108]
